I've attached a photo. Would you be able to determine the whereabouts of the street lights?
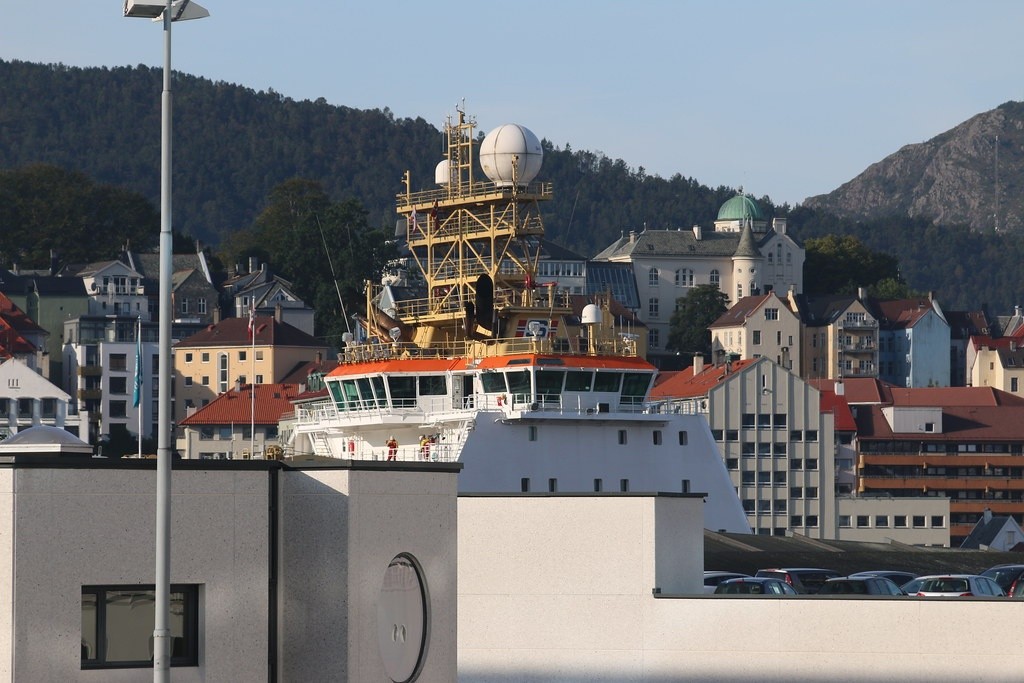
[123,0,212,683]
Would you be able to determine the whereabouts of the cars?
[702,563,1023,600]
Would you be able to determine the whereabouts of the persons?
[419,435,431,461]
[387,435,399,463]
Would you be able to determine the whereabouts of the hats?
[419,436,424,441]
[390,435,394,441]
